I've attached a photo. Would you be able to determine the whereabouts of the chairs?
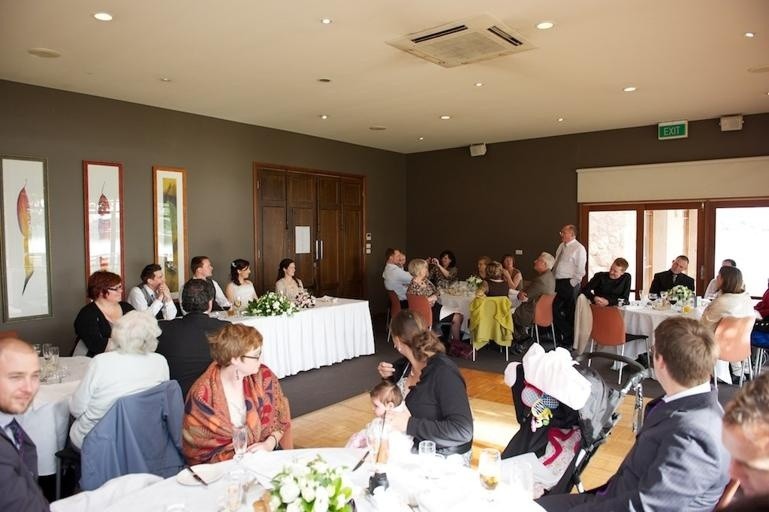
[751,329,769,377]
[587,304,651,385]
[713,479,740,511]
[714,317,756,389]
[56,380,187,499]
[386,288,556,361]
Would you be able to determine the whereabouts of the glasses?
[108,285,122,291]
[241,350,262,362]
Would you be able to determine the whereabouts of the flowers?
[666,285,694,301]
[242,291,299,316]
[465,276,483,288]
[294,291,316,310]
[266,453,354,512]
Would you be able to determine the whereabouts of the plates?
[176,464,222,486]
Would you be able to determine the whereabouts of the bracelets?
[270,432,281,451]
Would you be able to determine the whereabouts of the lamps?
[470,141,487,156]
[721,116,743,131]
[535,20,556,29]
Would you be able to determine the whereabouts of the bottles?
[368,434,389,498]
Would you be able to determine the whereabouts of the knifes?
[182,464,209,486]
[351,449,369,471]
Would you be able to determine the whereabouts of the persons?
[553,225,588,347]
[350,380,414,453]
[155,279,231,379]
[377,310,473,463]
[0,334,49,511]
[384,248,554,344]
[66,311,171,454]
[716,369,769,509]
[585,257,632,307]
[538,318,734,510]
[71,271,137,357]
[127,256,304,319]
[649,256,755,333]
[181,324,290,466]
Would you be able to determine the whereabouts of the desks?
[98,447,547,512]
[20,357,91,477]
[207,295,376,379]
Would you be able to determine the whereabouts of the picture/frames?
[0,155,53,326]
[153,166,189,304]
[84,160,126,305]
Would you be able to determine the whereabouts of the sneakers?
[717,373,754,384]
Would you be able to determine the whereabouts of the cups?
[683,300,690,312]
[48,348,59,367]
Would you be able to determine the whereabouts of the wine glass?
[443,280,460,294]
[233,424,249,477]
[223,302,231,320]
[648,293,657,311]
[660,292,668,306]
[669,296,678,313]
[638,290,645,305]
[419,441,437,479]
[43,343,53,369]
[479,449,501,502]
[234,296,242,318]
[32,344,42,360]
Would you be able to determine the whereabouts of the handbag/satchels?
[448,339,473,360]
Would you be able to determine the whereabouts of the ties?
[673,275,677,283]
[8,418,24,462]
[596,401,665,496]
[551,242,566,276]
